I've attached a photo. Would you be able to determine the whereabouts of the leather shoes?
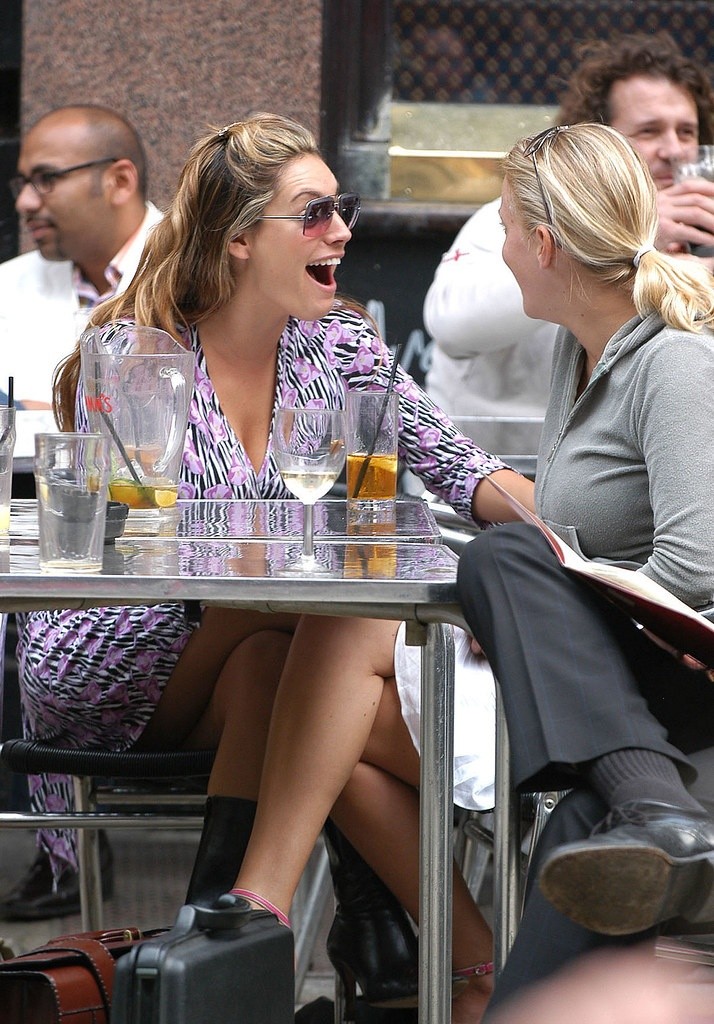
[0,826,113,917]
[537,796,714,936]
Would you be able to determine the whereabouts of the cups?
[0,404,17,537]
[668,145,714,257]
[344,391,400,512]
[33,431,112,574]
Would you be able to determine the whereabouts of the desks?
[0,497,516,1024]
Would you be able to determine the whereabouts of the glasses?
[256,192,362,238]
[8,156,123,195]
[524,124,568,251]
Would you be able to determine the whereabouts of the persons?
[230,122,714,1024]
[0,106,166,921]
[400,36,714,500]
[21,120,537,1001]
[453,524,714,1024]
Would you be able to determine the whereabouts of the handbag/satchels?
[0,925,167,1024]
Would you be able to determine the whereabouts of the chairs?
[0,736,218,933]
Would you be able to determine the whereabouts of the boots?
[318,819,466,1019]
[182,792,260,908]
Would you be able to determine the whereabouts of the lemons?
[348,457,397,472]
[152,490,178,506]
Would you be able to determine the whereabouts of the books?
[475,467,713,673]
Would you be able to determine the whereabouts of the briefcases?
[111,892,296,1022]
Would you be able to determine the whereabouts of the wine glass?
[273,407,348,577]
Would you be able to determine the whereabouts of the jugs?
[81,325,196,518]
